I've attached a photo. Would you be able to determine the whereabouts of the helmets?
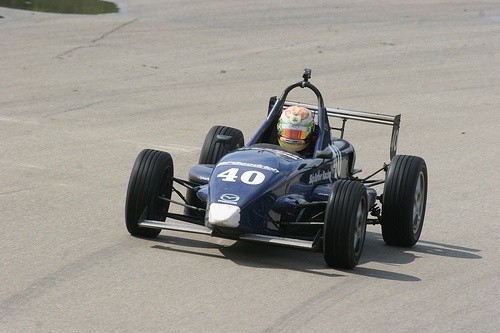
[277,105,315,151]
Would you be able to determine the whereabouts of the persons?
[260,105,319,160]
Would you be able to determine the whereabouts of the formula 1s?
[125,69,428,270]
